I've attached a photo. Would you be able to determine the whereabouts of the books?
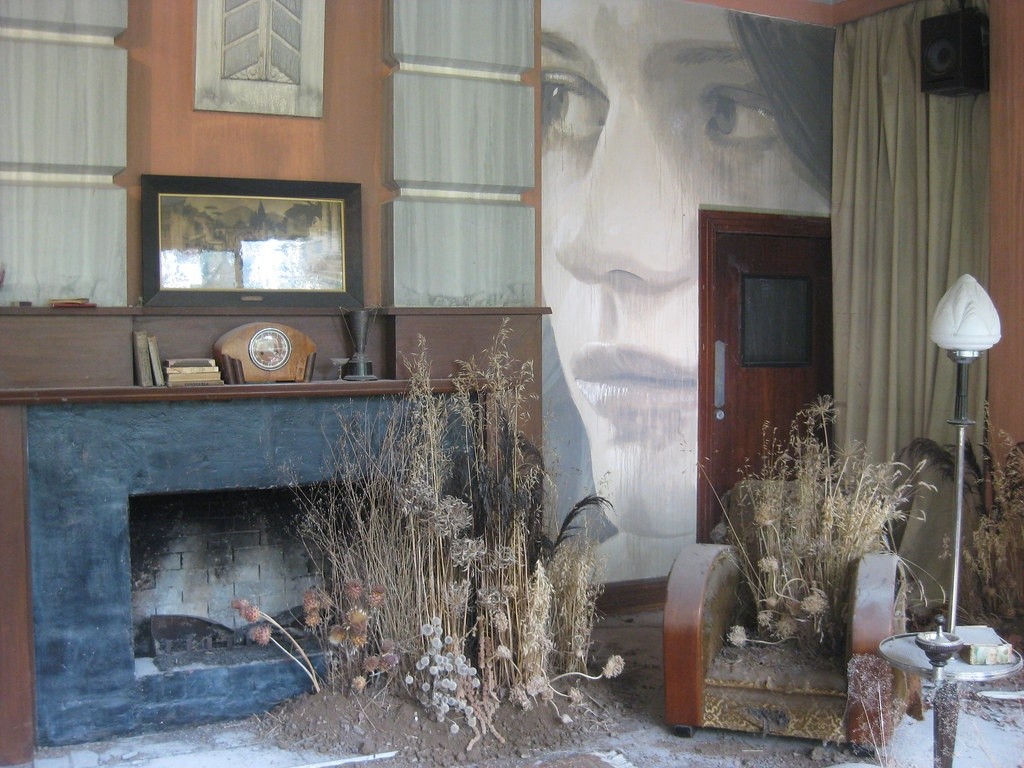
[49,299,97,308]
[133,330,224,387]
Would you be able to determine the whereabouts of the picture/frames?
[139,173,364,309]
[194,0,327,120]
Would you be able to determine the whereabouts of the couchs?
[658,478,928,759]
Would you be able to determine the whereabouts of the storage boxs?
[958,634,1014,667]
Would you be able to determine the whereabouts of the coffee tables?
[875,631,1024,768]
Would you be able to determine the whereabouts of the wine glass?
[329,358,349,382]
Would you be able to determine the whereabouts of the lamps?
[924,273,1003,630]
